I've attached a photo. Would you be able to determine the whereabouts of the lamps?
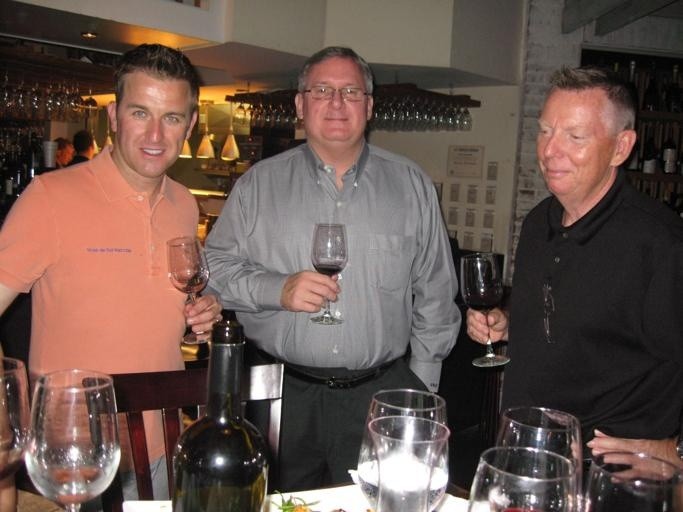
[179,127,246,168]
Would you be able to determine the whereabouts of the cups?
[466,403,683,512]
[44,140,59,169]
[0,356,33,483]
[355,386,449,512]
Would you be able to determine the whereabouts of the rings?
[212,316,220,324]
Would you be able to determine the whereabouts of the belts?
[262,352,392,389]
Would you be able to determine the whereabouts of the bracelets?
[675,432,682,460]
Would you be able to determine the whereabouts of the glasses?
[540,281,556,346]
[304,84,369,102]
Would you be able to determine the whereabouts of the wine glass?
[165,235,213,347]
[461,252,511,369]
[22,366,123,512]
[0,73,98,122]
[310,222,347,327]
[231,93,474,132]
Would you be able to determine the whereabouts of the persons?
[65,129,95,166]
[1,42,226,511]
[465,63,683,496]
[191,45,460,494]
[51,136,73,170]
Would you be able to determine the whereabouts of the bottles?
[0,129,44,208]
[170,318,271,512]
[607,57,683,219]
[367,414,453,512]
